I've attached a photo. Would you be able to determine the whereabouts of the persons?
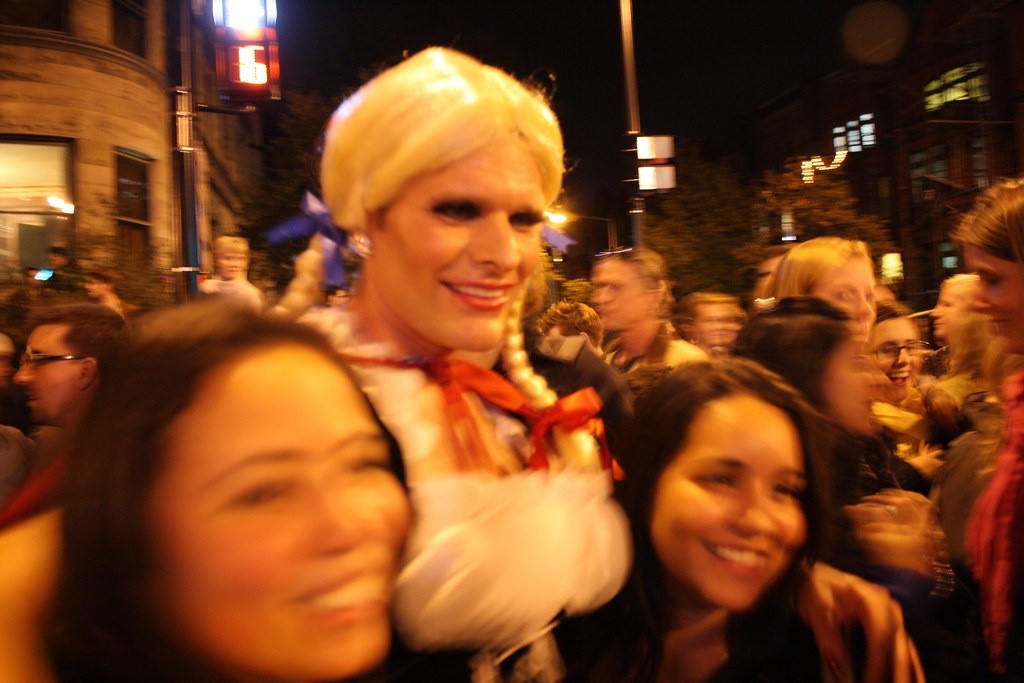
[1,46,926,683]
[580,360,895,683]
[2,230,340,529]
[40,298,469,683]
[525,178,1024,683]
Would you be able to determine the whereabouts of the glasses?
[19,352,86,367]
[866,341,929,361]
